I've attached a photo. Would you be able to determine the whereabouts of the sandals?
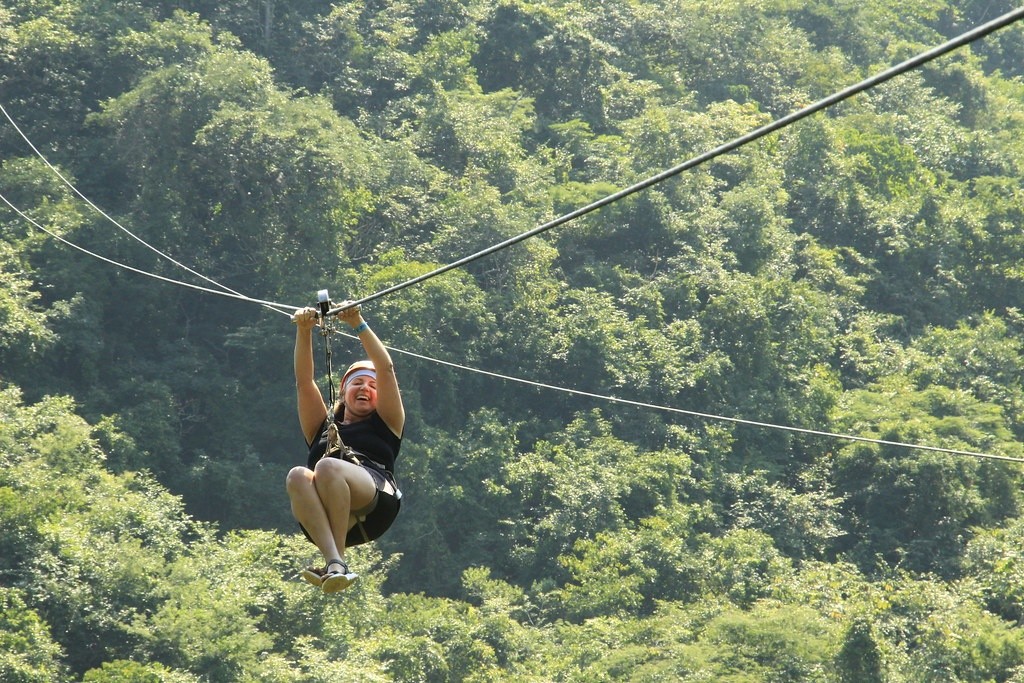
[306,560,361,594]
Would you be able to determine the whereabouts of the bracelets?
[354,322,367,334]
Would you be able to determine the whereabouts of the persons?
[287,300,405,592]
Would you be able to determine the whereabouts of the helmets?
[342,361,380,389]
